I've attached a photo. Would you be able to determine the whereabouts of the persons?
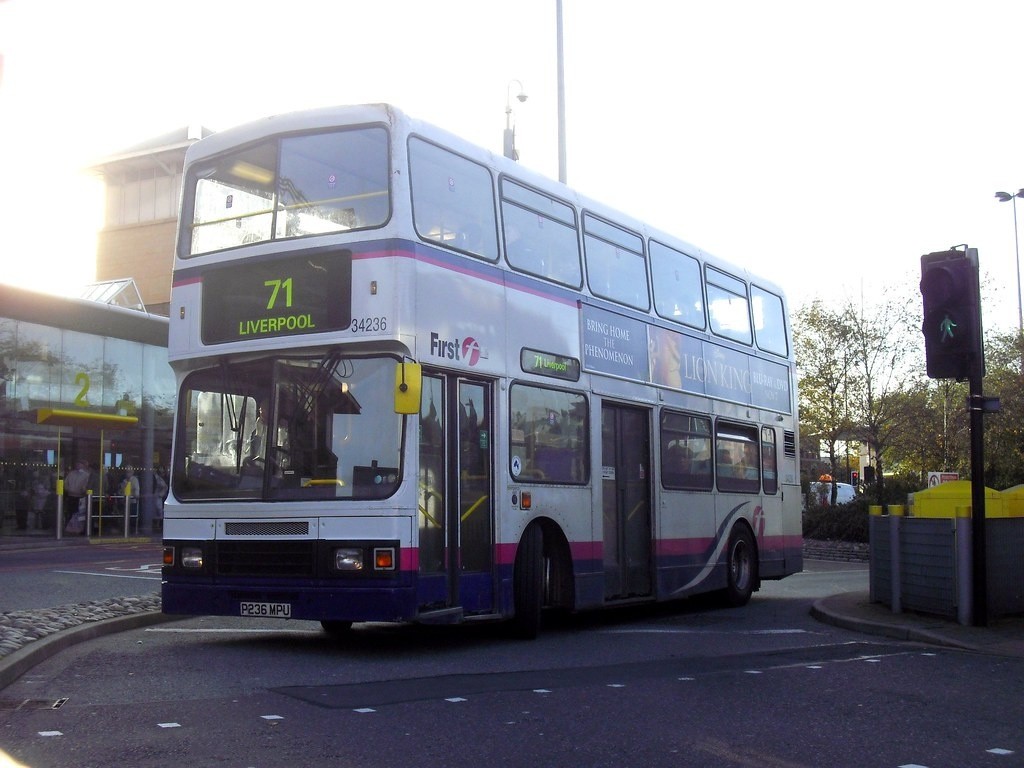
[667,443,770,474]
[248,399,289,467]
[530,426,585,482]
[15,458,168,530]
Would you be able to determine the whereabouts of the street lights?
[994,186,1024,372]
[501,78,528,165]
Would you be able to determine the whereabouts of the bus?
[159,100,806,638]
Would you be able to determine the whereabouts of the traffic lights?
[920,243,984,380]
[851,471,858,486]
